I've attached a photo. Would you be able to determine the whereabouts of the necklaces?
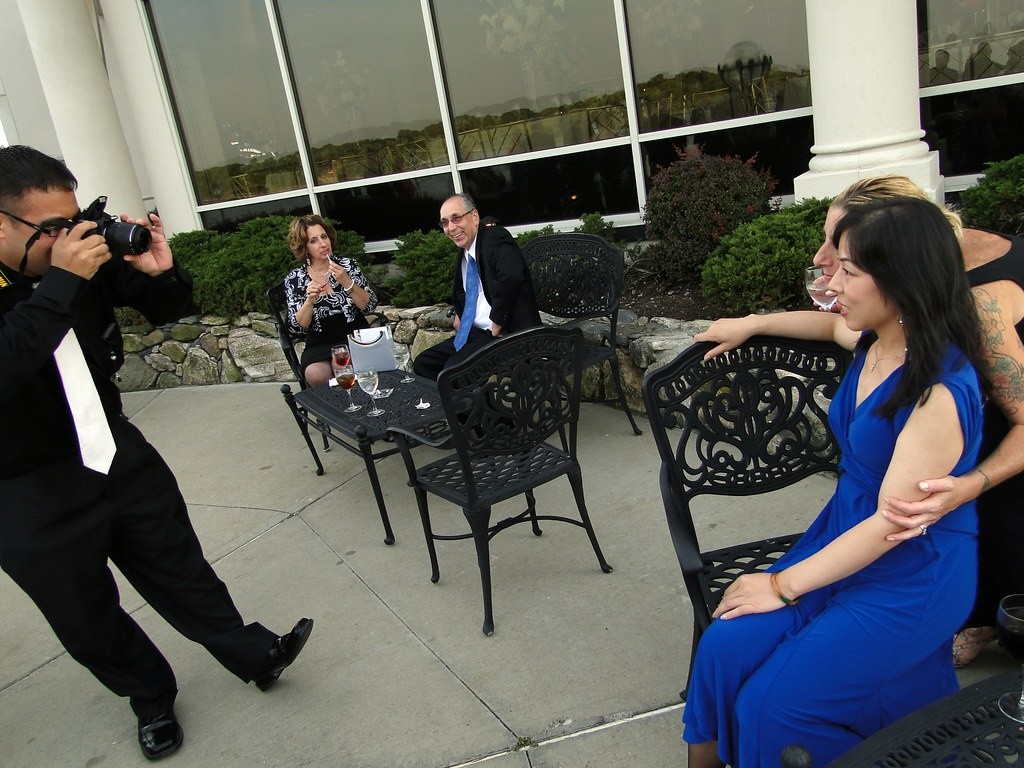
[870,341,905,371]
[309,265,330,278]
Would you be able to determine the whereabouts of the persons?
[414,193,543,440]
[812,175,1024,663]
[681,197,988,767]
[284,211,378,386]
[1,145,314,760]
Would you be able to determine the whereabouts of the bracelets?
[344,279,355,292]
[770,570,799,606]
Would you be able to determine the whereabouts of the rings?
[918,525,926,535]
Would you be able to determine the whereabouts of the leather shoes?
[252,618,314,692]
[137,707,183,760]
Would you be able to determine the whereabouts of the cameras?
[66,196,152,254]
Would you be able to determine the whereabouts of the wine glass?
[998,594,1024,722]
[393,344,415,383]
[333,364,362,412]
[356,367,385,417]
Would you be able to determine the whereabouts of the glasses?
[1,210,79,237]
[438,208,474,227]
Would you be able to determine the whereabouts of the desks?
[278,369,498,547]
[820,674,1024,768]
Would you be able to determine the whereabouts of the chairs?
[387,327,614,635]
[267,280,390,449]
[639,335,851,698]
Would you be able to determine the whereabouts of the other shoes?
[952,623,998,668]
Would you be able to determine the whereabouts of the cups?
[331,344,350,370]
[805,265,838,312]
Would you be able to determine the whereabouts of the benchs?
[445,234,643,458]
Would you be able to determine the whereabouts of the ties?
[452,253,479,351]
[32,282,117,475]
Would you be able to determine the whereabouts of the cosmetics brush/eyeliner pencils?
[325,253,333,265]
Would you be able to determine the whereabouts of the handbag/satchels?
[347,326,398,374]
